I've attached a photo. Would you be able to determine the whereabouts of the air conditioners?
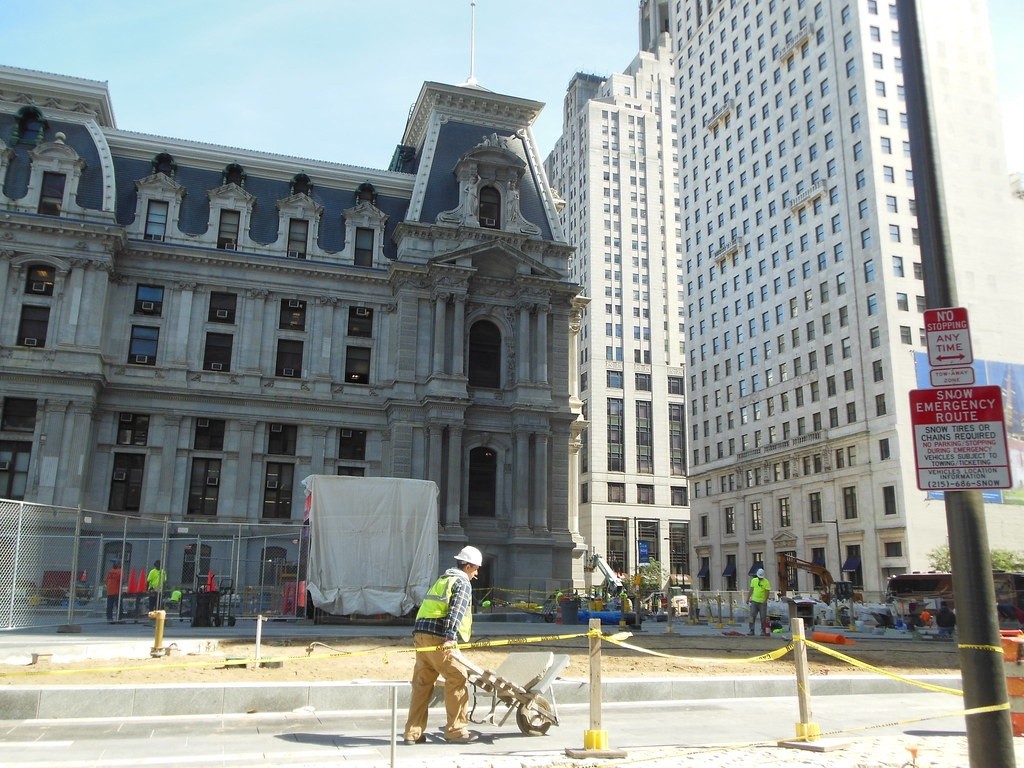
[152,233,163,242]
[136,355,147,363]
[356,308,366,316]
[267,480,278,489]
[288,251,299,258]
[342,430,352,438]
[198,418,209,427]
[113,472,125,480]
[217,309,227,317]
[486,218,495,226]
[288,300,299,307]
[226,243,236,251]
[121,413,132,421]
[142,301,153,311]
[212,363,222,370]
[25,337,37,346]
[283,368,294,376]
[271,424,282,432]
[33,282,45,291]
[0,461,9,470]
[207,477,218,485]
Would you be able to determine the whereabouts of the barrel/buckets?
[999,629,1024,738]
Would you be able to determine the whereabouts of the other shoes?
[747,630,756,635]
[760,631,765,634]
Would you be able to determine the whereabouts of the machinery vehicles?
[778,553,864,605]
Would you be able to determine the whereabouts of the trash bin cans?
[190,591,220,627]
[559,600,578,624]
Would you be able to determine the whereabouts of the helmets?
[114,560,121,568]
[454,546,482,568]
[757,569,765,578]
[154,559,160,567]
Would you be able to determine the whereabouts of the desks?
[787,601,814,632]
[116,591,173,623]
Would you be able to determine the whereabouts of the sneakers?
[404,734,426,745]
[447,732,479,743]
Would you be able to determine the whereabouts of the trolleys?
[449,649,559,736]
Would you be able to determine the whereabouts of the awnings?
[807,558,825,574]
[722,565,736,577]
[696,565,709,578]
[842,555,861,573]
[748,561,763,577]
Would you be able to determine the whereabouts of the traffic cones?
[79,569,87,583]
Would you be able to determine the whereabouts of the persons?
[105,558,125,624]
[746,569,770,636]
[404,546,482,745]
[146,559,167,612]
[921,600,956,637]
[554,588,700,618]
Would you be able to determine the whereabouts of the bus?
[886,570,1024,627]
